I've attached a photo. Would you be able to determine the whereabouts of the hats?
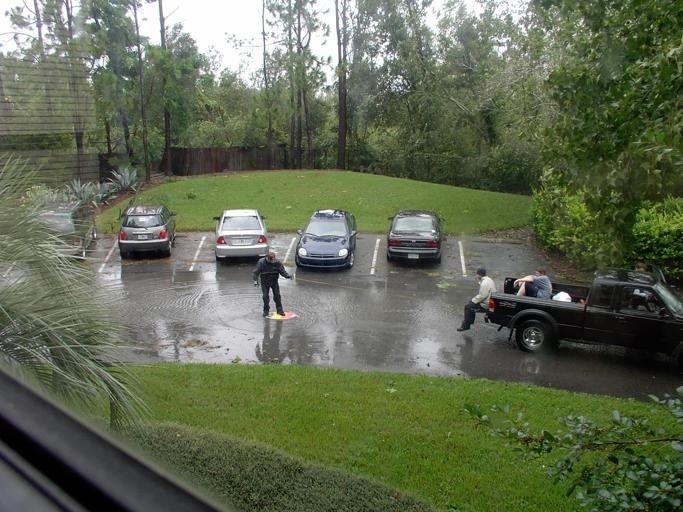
[477,269,486,276]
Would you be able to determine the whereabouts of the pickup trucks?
[472,274,681,366]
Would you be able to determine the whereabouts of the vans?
[37,199,96,261]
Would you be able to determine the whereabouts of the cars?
[215,210,268,264]
[295,206,358,273]
[384,208,445,264]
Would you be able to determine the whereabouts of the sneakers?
[457,327,470,331]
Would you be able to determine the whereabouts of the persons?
[456,268,495,332]
[251,251,294,318]
[633,263,646,273]
[513,266,553,300]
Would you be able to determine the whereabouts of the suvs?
[118,205,178,258]
